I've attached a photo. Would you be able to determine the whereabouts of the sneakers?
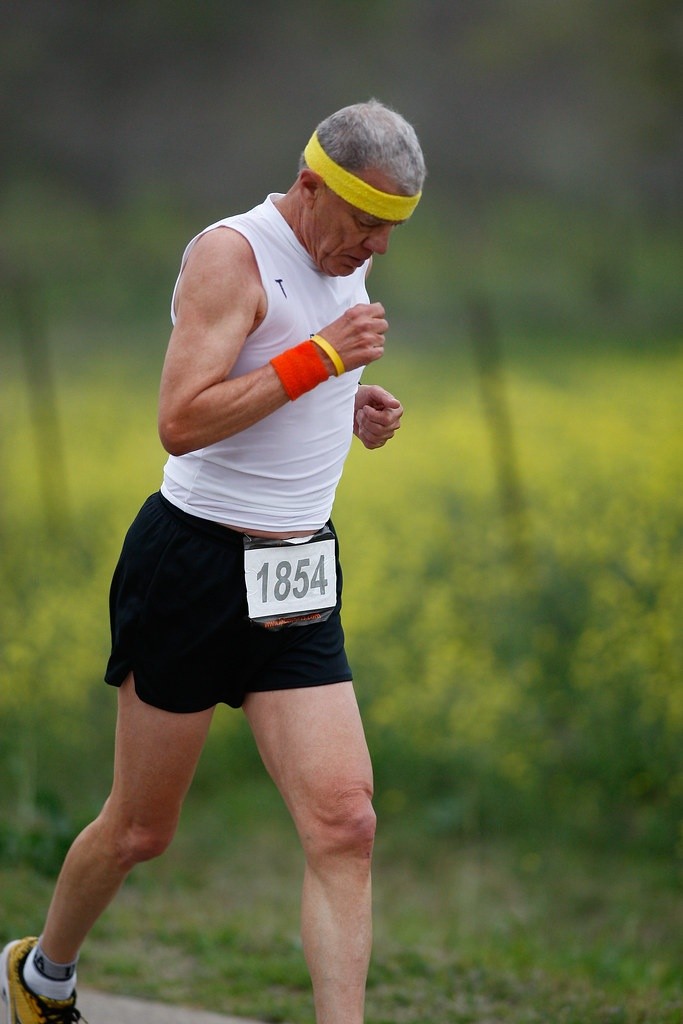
[0,936,88,1024]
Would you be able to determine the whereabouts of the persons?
[1,99,426,1022]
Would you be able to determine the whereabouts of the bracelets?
[310,335,345,377]
[268,341,330,401]
